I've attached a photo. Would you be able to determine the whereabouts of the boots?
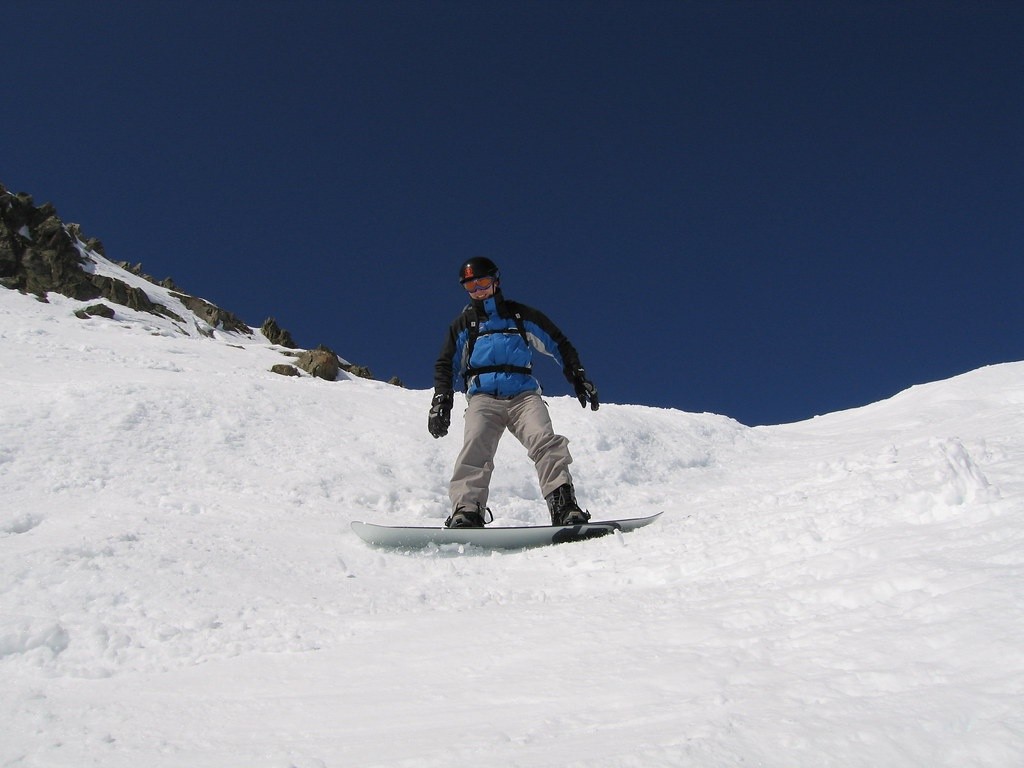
[450,512,485,528]
[545,484,588,526]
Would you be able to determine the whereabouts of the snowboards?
[350,510,665,550]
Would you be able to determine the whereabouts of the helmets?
[459,257,498,283]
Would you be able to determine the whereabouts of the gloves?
[566,368,599,411]
[428,391,455,439]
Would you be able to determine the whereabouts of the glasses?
[462,271,500,293]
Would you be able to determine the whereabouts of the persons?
[427,258,599,527]
